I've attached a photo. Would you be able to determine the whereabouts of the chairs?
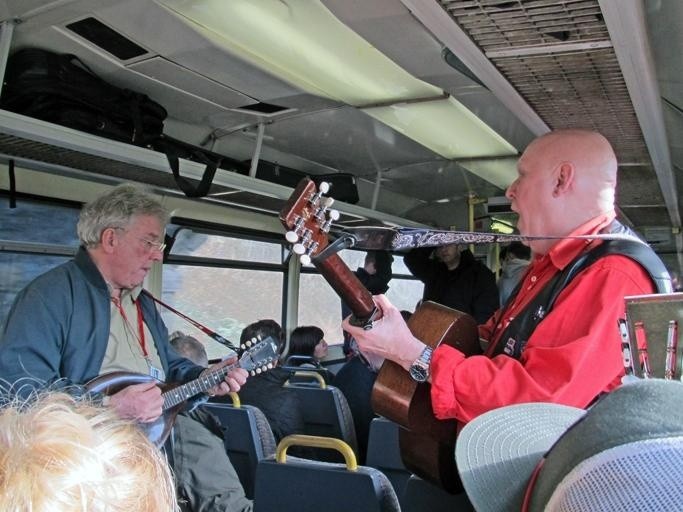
[179,349,416,512]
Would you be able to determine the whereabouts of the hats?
[454,379,682,512]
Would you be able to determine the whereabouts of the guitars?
[81,331,281,450]
[276,175,484,493]
